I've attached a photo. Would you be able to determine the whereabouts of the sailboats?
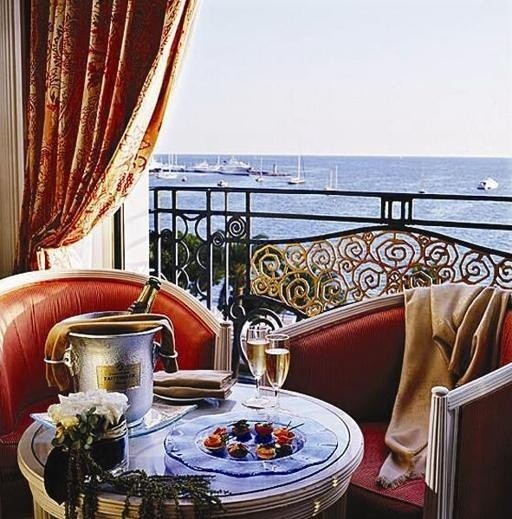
[417,158,429,194]
[325,163,344,191]
[288,151,306,187]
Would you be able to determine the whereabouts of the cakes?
[204,421,296,459]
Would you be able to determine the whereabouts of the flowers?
[44,389,129,455]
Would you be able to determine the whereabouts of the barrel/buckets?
[43,310,178,428]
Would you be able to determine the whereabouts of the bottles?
[126,277,161,314]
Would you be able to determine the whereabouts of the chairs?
[1,265,236,519]
[260,285,510,517]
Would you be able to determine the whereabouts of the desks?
[15,376,367,519]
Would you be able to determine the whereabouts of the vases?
[69,417,133,484]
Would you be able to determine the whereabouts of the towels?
[43,310,179,391]
[151,379,239,399]
[149,365,236,389]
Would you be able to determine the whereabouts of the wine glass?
[241,326,271,408]
[263,333,291,412]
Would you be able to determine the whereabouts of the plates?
[30,403,198,439]
[166,411,339,476]
[153,393,207,403]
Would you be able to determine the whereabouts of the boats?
[477,178,498,191]
[149,143,291,187]
